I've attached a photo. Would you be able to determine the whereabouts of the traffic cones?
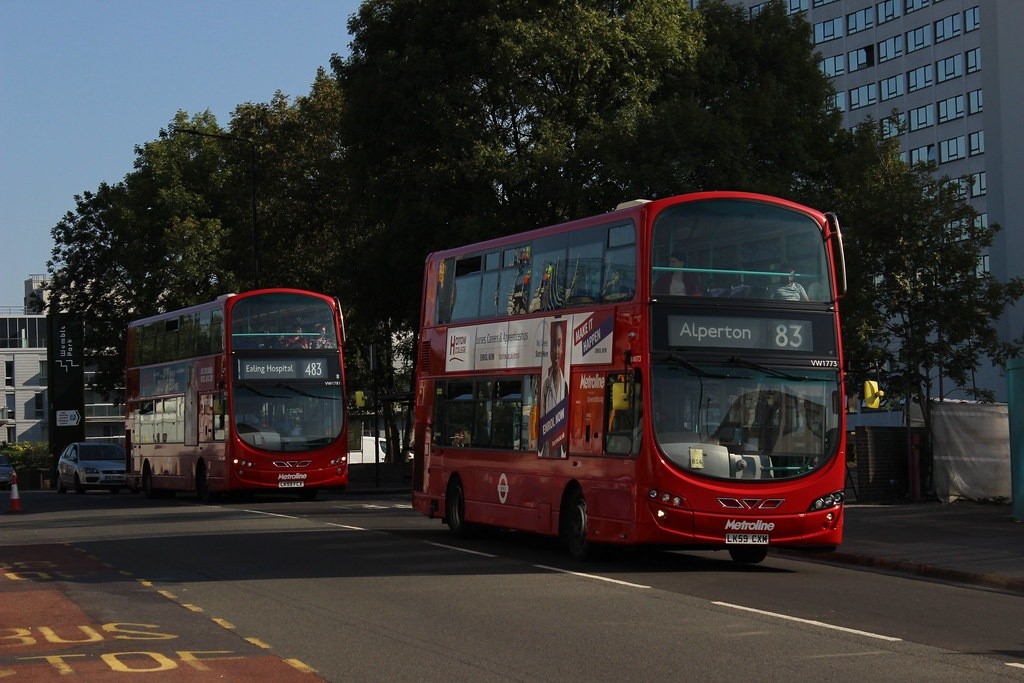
[9,472,22,512]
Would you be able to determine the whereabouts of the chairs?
[704,281,770,298]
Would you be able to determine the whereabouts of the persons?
[288,324,309,349]
[236,404,256,426]
[542,321,568,457]
[771,263,810,301]
[309,325,332,350]
[655,252,703,296]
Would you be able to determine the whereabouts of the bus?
[412,191,884,564]
[124,288,368,501]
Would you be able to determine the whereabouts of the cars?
[0,454,16,490]
[56,442,127,496]
